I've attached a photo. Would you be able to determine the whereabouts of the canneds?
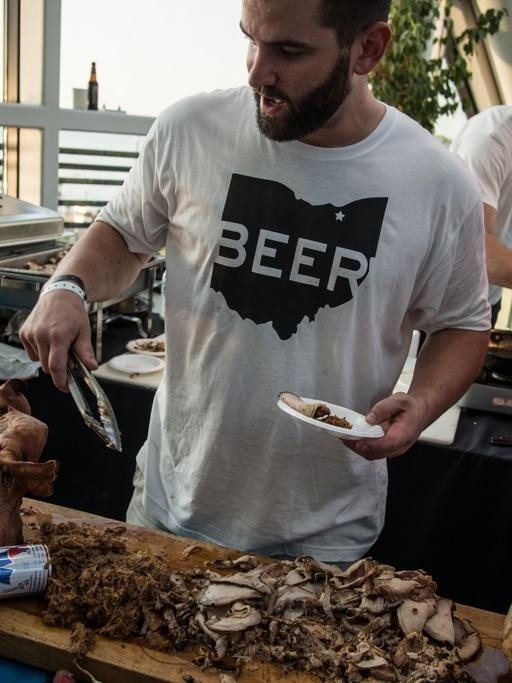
[1,541,52,599]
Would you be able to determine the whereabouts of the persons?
[18,0,493,573]
[415,105,512,359]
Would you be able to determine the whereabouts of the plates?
[126,338,166,357]
[108,354,165,374]
[277,397,385,441]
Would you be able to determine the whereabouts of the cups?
[73,88,88,111]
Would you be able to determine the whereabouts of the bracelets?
[37,282,86,302]
[43,275,88,300]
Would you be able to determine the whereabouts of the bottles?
[87,62,99,111]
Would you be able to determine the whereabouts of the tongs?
[65,350,123,452]
